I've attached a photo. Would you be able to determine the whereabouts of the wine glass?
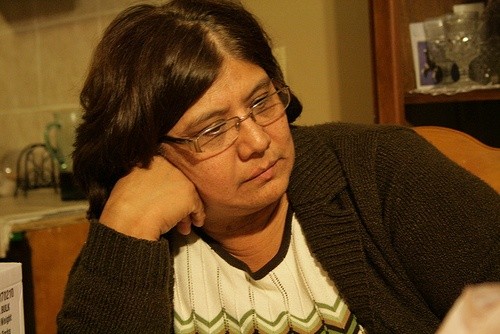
[424,15,455,93]
[444,12,486,91]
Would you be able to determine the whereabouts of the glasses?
[161,79,290,152]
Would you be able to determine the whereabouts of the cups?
[45,109,85,201]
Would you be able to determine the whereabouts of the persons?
[55,0,500,334]
